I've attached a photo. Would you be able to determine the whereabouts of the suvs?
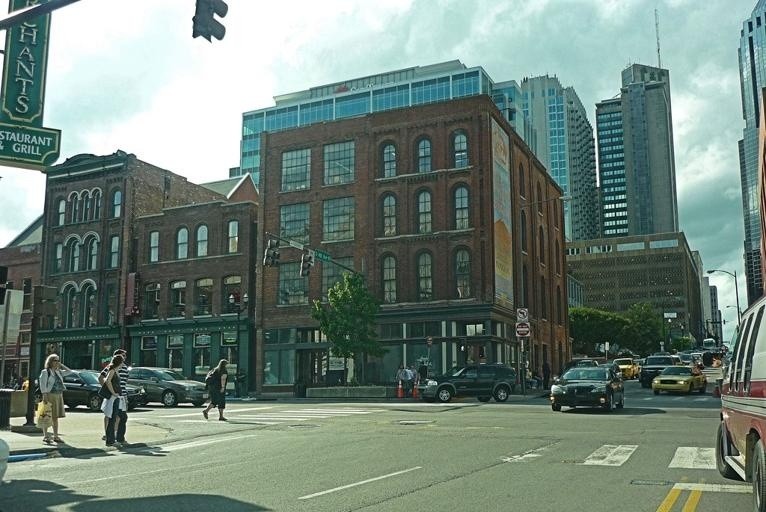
[40,368,141,411]
[124,366,210,408]
[423,362,518,402]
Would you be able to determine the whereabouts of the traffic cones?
[412,380,418,397]
[396,379,404,398]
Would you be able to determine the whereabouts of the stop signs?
[516,322,530,337]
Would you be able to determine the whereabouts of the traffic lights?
[262,239,280,267]
[194,1,227,44]
[301,254,311,276]
[458,338,465,351]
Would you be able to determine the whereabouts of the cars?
[550,338,730,411]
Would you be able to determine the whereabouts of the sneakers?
[203,409,210,420]
[42,435,63,444]
[105,438,130,448]
[218,417,227,421]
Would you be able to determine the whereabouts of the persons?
[393,359,428,397]
[98,347,129,441]
[100,353,129,448]
[526,357,551,390]
[202,358,229,421]
[37,351,69,445]
[11,372,29,391]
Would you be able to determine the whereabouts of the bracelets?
[222,386,225,389]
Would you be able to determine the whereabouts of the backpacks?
[205,367,222,388]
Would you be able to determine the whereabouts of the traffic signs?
[314,248,330,263]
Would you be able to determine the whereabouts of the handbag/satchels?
[35,378,45,397]
[97,380,115,400]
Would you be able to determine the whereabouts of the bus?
[714,293,766,512]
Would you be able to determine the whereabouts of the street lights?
[667,319,673,352]
[229,291,250,397]
[680,324,685,351]
[726,305,737,308]
[708,269,742,326]
